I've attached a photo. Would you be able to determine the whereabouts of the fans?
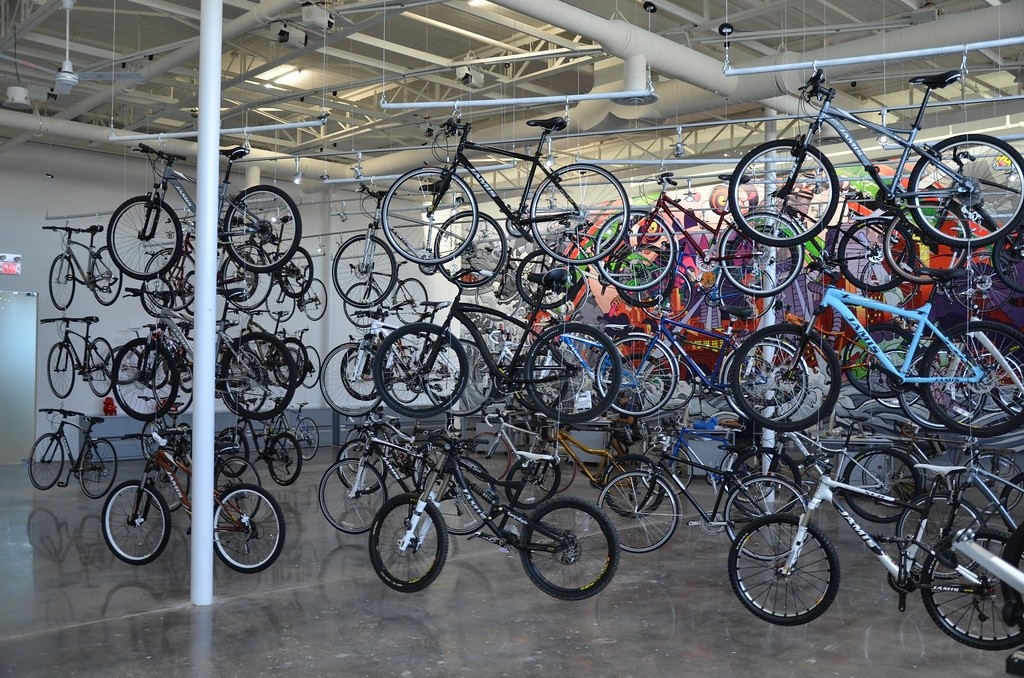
[0,1,142,95]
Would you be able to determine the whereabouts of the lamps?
[544,137,554,168]
[3,86,32,109]
[339,201,348,222]
[877,109,889,145]
[354,153,363,179]
[673,127,685,157]
[293,156,303,184]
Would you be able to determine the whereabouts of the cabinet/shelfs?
[78,407,451,458]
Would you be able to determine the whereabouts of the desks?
[463,408,895,496]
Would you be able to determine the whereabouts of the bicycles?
[105,139,303,279]
[367,430,623,603]
[26,407,118,500]
[41,222,123,311]
[98,424,287,574]
[380,112,631,267]
[40,315,116,399]
[103,167,1024,678]
[728,63,1023,250]
[261,396,320,462]
